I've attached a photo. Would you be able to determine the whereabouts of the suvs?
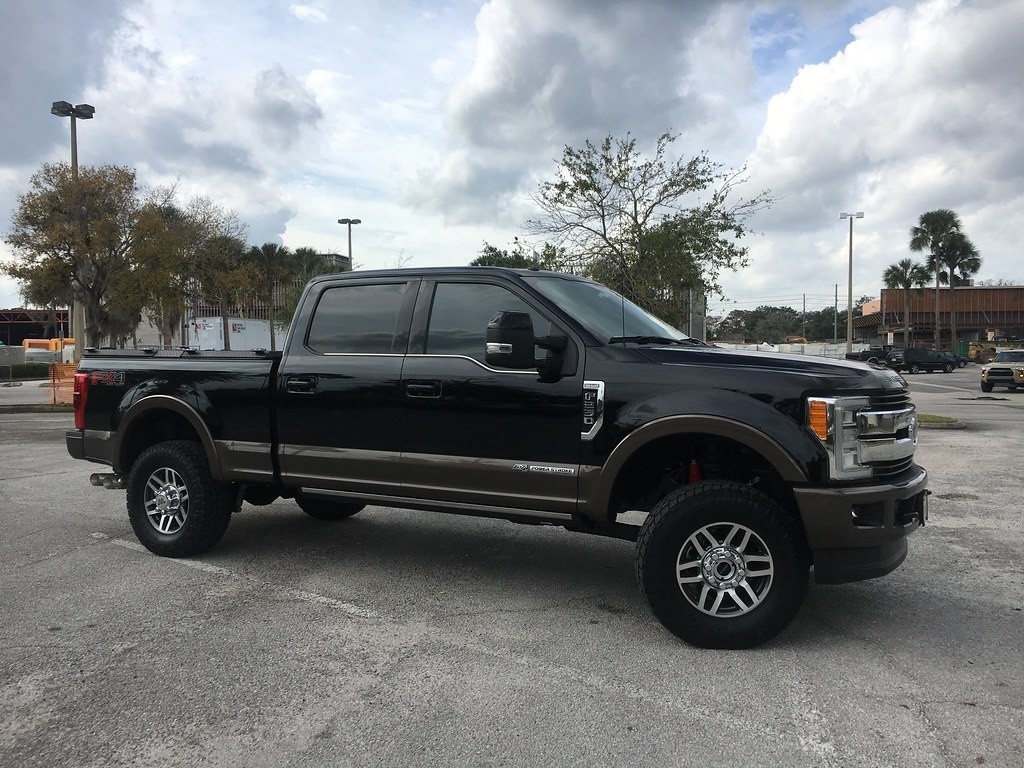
[845,344,968,374]
[980,349,1024,392]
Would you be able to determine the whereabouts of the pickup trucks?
[63,265,932,655]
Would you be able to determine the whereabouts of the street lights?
[338,218,361,271]
[51,100,97,362]
[839,211,865,356]
